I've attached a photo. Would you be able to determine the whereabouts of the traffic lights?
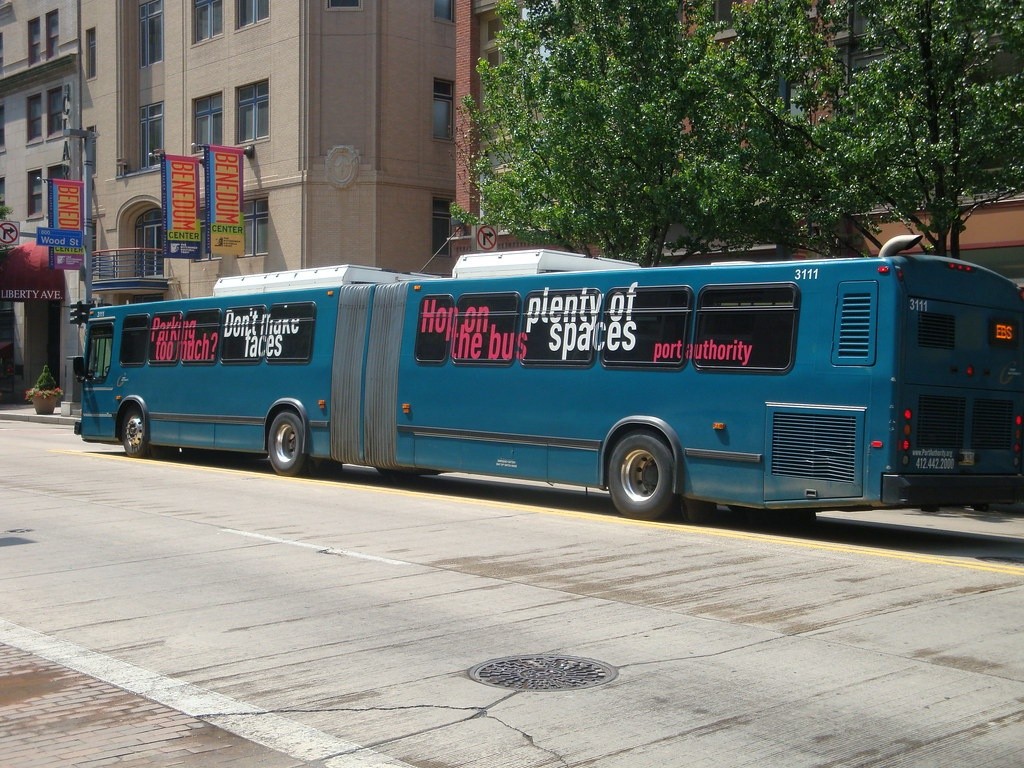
[69,301,82,325]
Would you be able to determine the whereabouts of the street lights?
[63,128,93,304]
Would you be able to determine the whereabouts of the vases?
[30,396,59,415]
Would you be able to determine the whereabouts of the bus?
[74,235,1024,520]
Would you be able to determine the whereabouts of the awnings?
[0,239,66,300]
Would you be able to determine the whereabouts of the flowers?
[25,365,64,402]
